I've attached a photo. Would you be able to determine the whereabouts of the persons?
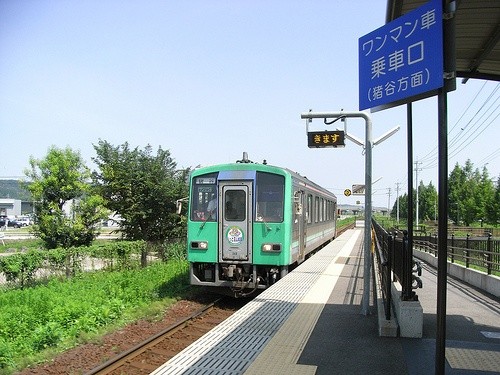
[207,199,217,220]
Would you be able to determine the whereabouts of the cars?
[0,214,31,228]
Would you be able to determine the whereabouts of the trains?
[175,152,337,299]
